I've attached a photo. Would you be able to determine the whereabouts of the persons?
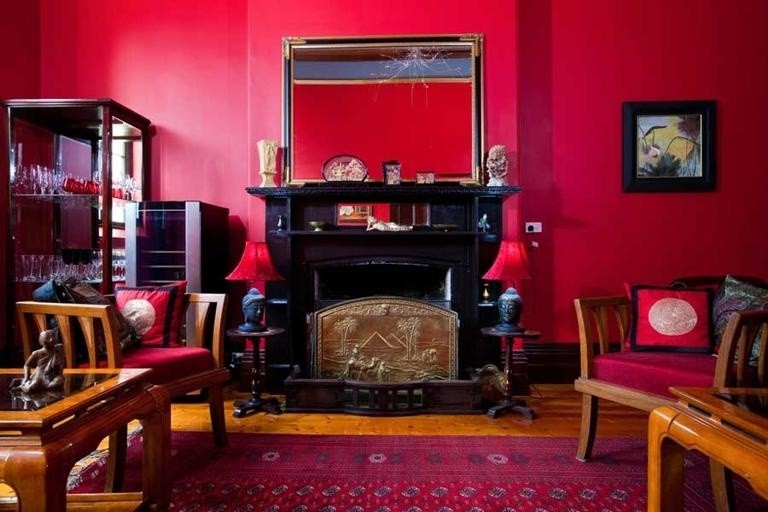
[11,329,66,394]
[366,216,414,232]
[239,288,267,332]
[494,287,526,333]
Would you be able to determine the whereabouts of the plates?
[322,155,368,182]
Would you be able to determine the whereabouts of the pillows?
[623,284,713,355]
[114,281,187,348]
[712,272,768,367]
[65,283,141,357]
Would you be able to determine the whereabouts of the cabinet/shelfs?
[124,198,228,294]
[5,97,154,359]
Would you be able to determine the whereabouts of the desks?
[224,327,284,417]
[645,384,766,511]
[480,327,540,419]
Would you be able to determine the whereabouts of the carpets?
[64,431,768,511]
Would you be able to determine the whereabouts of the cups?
[417,171,435,184]
[383,164,402,184]
[21,254,127,282]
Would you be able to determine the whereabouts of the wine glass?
[10,164,141,200]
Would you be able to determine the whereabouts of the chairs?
[575,280,766,512]
[16,281,231,494]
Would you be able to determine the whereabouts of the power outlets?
[526,222,543,233]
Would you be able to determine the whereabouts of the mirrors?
[281,36,483,187]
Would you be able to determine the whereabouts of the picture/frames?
[621,97,720,194]
